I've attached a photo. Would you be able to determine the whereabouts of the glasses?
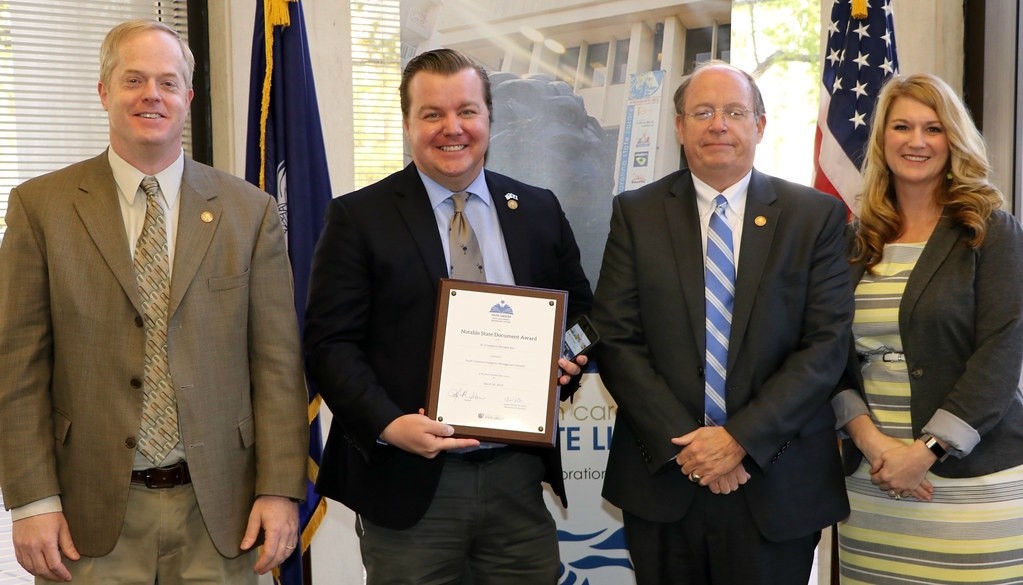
[686,109,754,120]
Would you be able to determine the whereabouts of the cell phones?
[563,314,600,363]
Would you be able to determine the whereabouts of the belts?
[863,352,905,362]
[131,461,192,489]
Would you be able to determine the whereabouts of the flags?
[807,0,906,225]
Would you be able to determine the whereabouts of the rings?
[690,472,702,486]
[889,489,901,500]
[285,546,296,551]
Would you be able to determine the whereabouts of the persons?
[586,58,852,585]
[836,75,1023,585]
[0,14,310,585]
[304,48,605,585]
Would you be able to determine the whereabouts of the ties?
[704,195,737,427]
[449,192,487,284]
[132,178,181,466]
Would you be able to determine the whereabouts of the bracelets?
[918,432,949,464]
